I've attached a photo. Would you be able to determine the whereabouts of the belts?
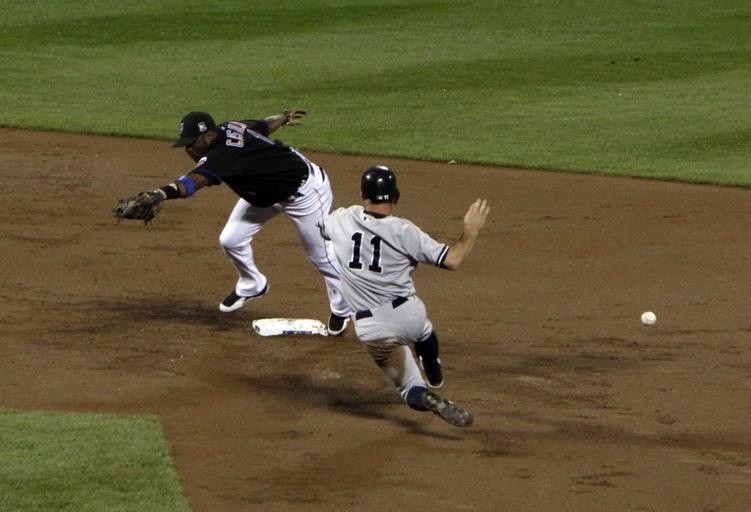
[356,296,409,320]
[280,158,314,207]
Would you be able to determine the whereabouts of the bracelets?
[283,110,290,126]
[156,182,181,200]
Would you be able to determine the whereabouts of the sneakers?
[421,390,474,427]
[327,312,351,336]
[218,279,270,313]
[419,355,444,389]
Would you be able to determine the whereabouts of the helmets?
[171,111,216,148]
[361,164,400,204]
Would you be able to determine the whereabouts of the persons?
[113,105,352,337]
[316,165,491,427]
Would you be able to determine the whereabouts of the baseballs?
[641,311,656,326]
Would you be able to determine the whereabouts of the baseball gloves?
[113,190,164,225]
